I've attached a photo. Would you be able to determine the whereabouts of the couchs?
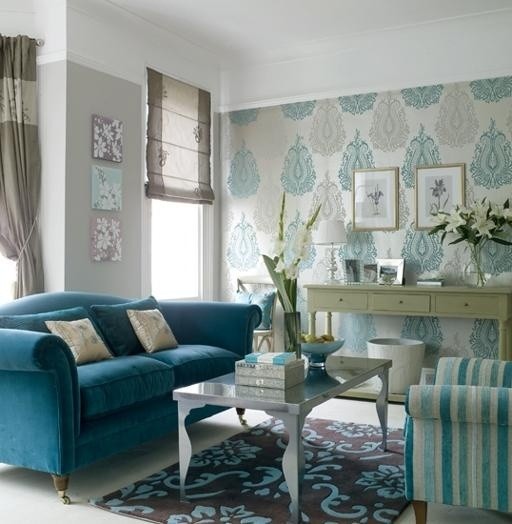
[0,290,261,505]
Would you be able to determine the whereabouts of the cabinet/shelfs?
[300,284,510,367]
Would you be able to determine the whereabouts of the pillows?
[1,296,180,363]
[235,288,275,330]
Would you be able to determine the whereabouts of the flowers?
[429,196,512,286]
[260,190,324,357]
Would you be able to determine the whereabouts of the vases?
[460,253,492,287]
[283,311,301,359]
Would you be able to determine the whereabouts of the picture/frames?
[349,162,466,231]
[345,258,404,286]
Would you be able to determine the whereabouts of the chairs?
[402,357,511,524]
[235,277,278,351]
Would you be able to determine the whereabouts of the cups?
[366,337,427,394]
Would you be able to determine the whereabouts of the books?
[245,351,297,364]
[417,279,446,287]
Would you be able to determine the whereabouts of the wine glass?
[302,365,343,400]
[298,337,346,368]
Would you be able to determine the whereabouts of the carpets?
[88,417,410,523]
